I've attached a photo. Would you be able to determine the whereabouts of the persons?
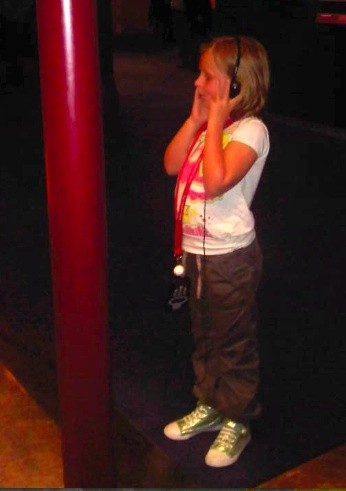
[162,35,272,469]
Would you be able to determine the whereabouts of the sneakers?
[203,417,252,469]
[163,400,221,440]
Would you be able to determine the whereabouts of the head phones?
[228,34,240,98]
